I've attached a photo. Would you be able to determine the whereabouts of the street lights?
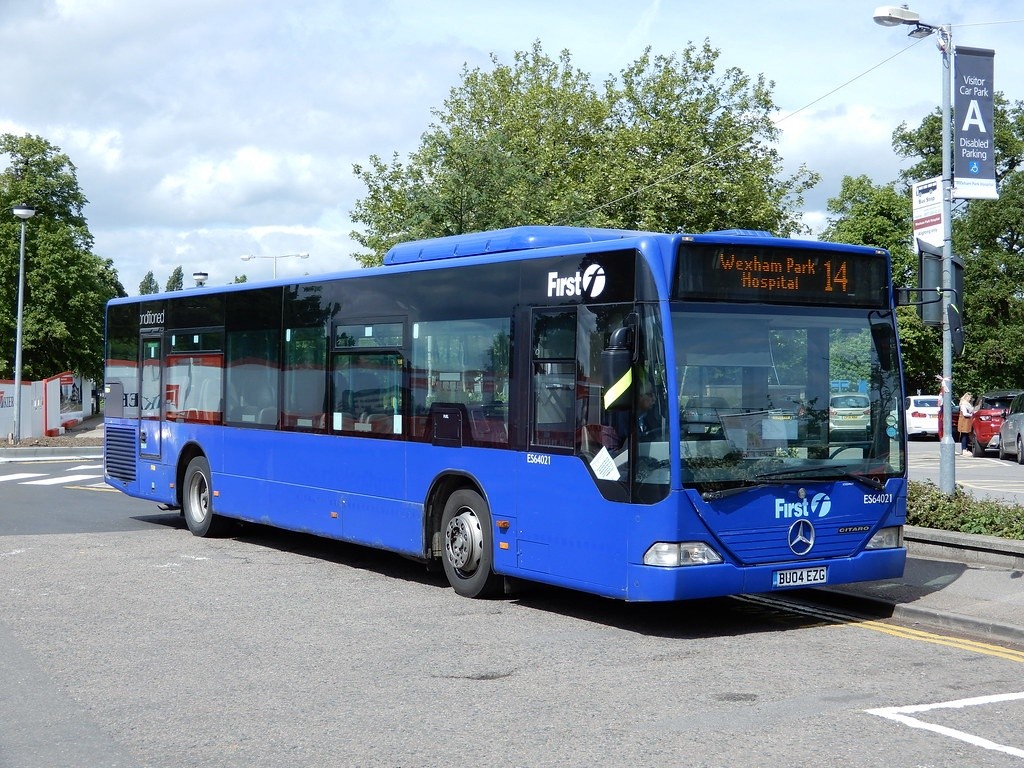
[873,5,956,499]
[240,252,308,279]
[12,202,38,445]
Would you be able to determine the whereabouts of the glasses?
[968,393,973,395]
[641,392,653,398]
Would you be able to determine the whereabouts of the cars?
[885,394,974,441]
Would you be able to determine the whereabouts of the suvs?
[967,389,1024,464]
[830,391,869,448]
[638,395,804,444]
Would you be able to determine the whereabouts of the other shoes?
[963,449,973,456]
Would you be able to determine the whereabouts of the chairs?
[233,403,509,443]
[686,396,732,411]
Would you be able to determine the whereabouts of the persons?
[634,393,658,442]
[958,392,978,456]
[937,387,960,455]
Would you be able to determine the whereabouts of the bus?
[105,225,965,603]
